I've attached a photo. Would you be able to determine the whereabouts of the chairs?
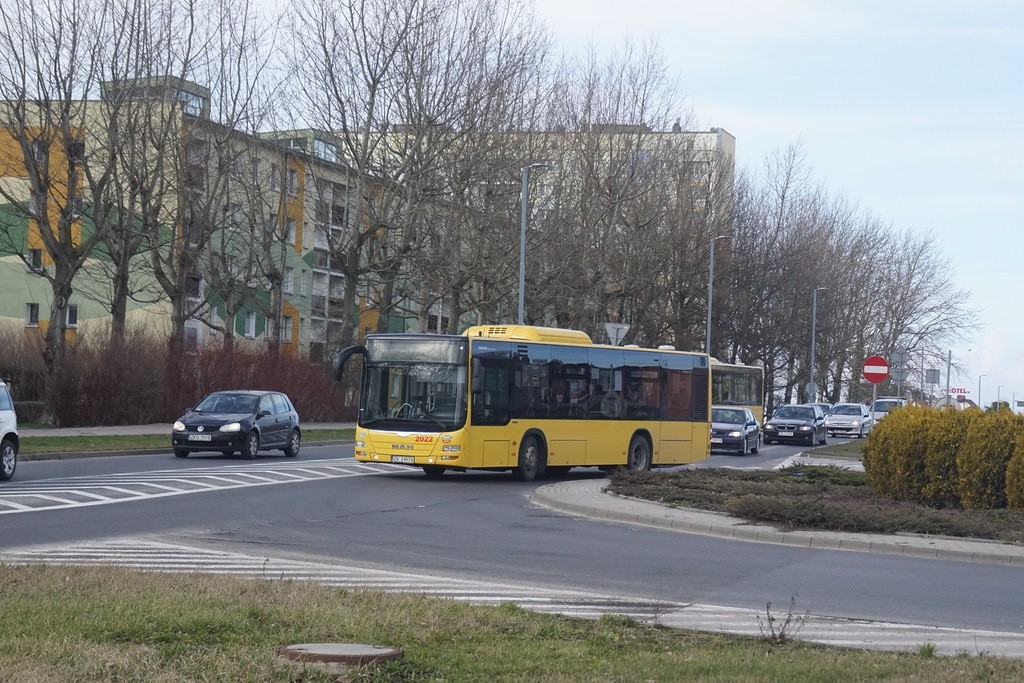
[482,390,690,417]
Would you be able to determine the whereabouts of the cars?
[0,378,19,481]
[710,406,761,455]
[805,394,907,439]
[762,404,828,446]
[173,390,304,457]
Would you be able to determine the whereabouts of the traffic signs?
[862,356,888,384]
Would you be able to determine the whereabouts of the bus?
[330,324,713,487]
[712,357,766,431]
